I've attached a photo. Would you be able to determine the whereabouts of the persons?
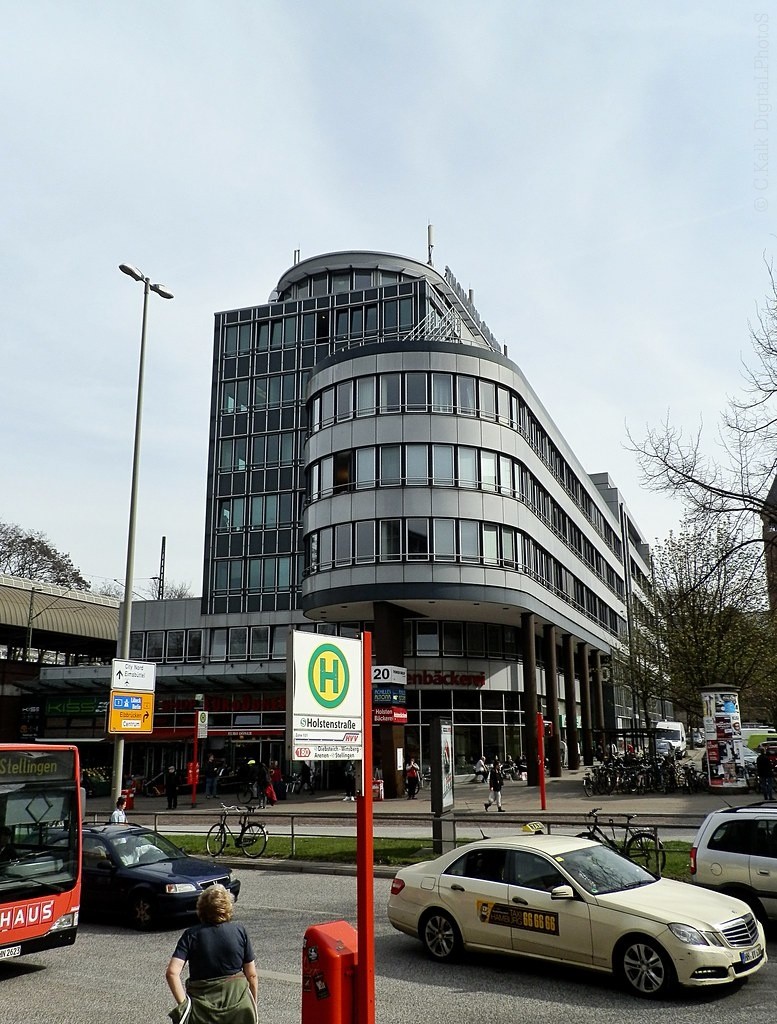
[123,836,163,866]
[110,797,129,826]
[164,765,179,810]
[475,754,528,785]
[484,761,506,812]
[294,760,315,796]
[343,760,357,801]
[757,747,776,800]
[722,695,736,713]
[597,738,617,764]
[165,884,258,1024]
[405,758,420,801]
[204,752,284,809]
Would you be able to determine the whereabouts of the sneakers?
[343,796,350,801]
[351,796,354,801]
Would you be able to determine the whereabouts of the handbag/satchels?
[266,784,277,806]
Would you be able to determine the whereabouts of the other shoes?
[166,806,176,810]
[213,795,219,797]
[407,797,411,799]
[207,796,210,798]
[294,790,299,794]
[484,804,489,811]
[497,808,506,812]
[412,797,417,799]
[309,792,314,795]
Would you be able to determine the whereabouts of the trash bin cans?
[121,789,134,810]
[372,780,384,801]
[302,921,359,1024]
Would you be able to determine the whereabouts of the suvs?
[22,821,241,932]
[691,800,777,930]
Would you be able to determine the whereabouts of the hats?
[247,760,256,764]
[169,766,175,768]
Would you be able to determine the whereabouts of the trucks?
[656,722,686,756]
[742,729,777,750]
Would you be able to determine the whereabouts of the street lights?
[110,261,175,812]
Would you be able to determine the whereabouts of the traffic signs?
[108,690,155,735]
[110,658,156,690]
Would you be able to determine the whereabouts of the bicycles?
[237,783,257,804]
[575,807,667,875]
[206,803,269,859]
[752,761,777,799]
[583,755,708,797]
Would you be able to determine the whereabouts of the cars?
[686,728,706,748]
[388,822,768,1000]
[702,741,777,776]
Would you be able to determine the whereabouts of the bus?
[0,744,86,959]
